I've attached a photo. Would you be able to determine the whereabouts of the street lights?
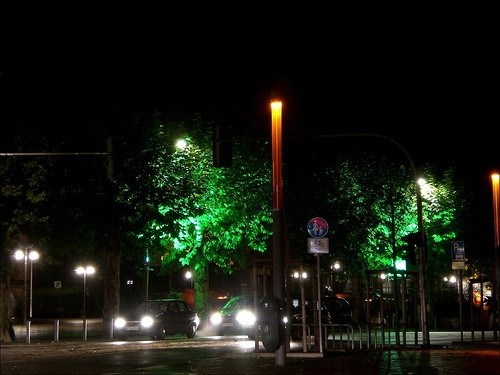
[13,244,40,345]
[293,264,308,354]
[415,177,430,348]
[74,263,96,343]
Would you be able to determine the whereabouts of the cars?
[113,298,202,342]
[200,293,354,353]
